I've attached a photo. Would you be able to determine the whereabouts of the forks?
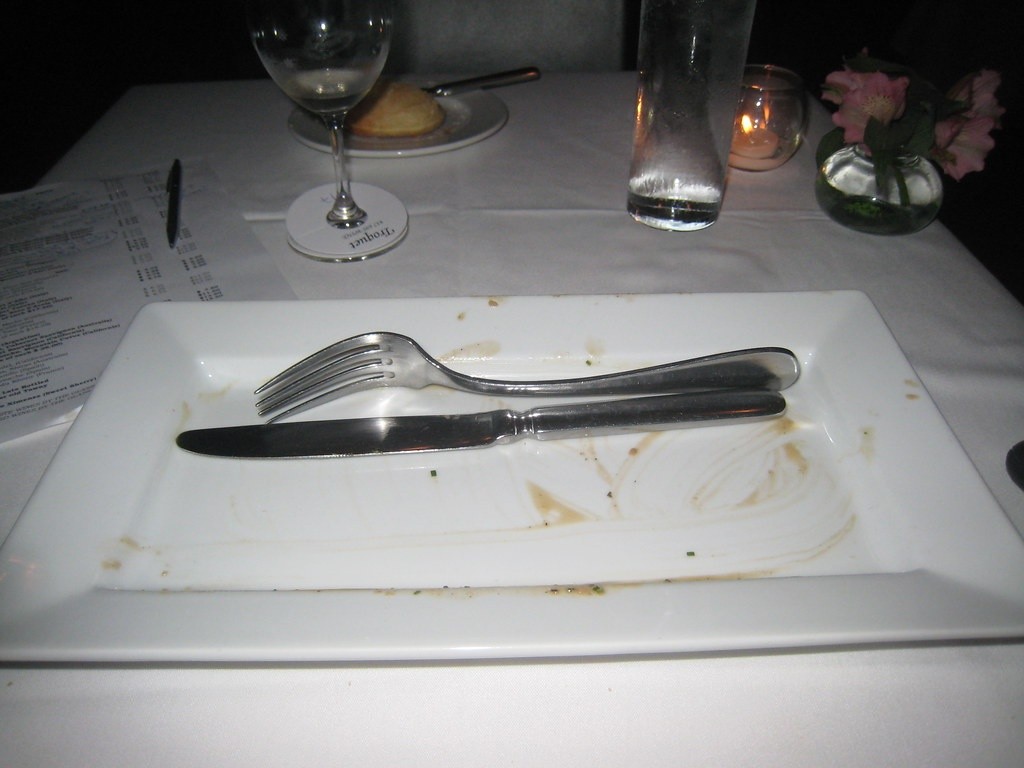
[252,330,800,436]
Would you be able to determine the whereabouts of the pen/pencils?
[164,158,182,248]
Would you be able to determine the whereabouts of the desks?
[0,70,1023,767]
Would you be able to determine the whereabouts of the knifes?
[175,388,787,459]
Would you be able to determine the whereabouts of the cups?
[625,0,763,232]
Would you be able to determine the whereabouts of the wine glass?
[243,0,410,262]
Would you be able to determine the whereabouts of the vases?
[814,143,945,236]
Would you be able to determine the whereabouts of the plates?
[0,289,1024,665]
[286,79,510,159]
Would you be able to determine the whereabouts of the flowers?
[816,41,1006,183]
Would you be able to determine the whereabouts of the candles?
[730,115,779,159]
